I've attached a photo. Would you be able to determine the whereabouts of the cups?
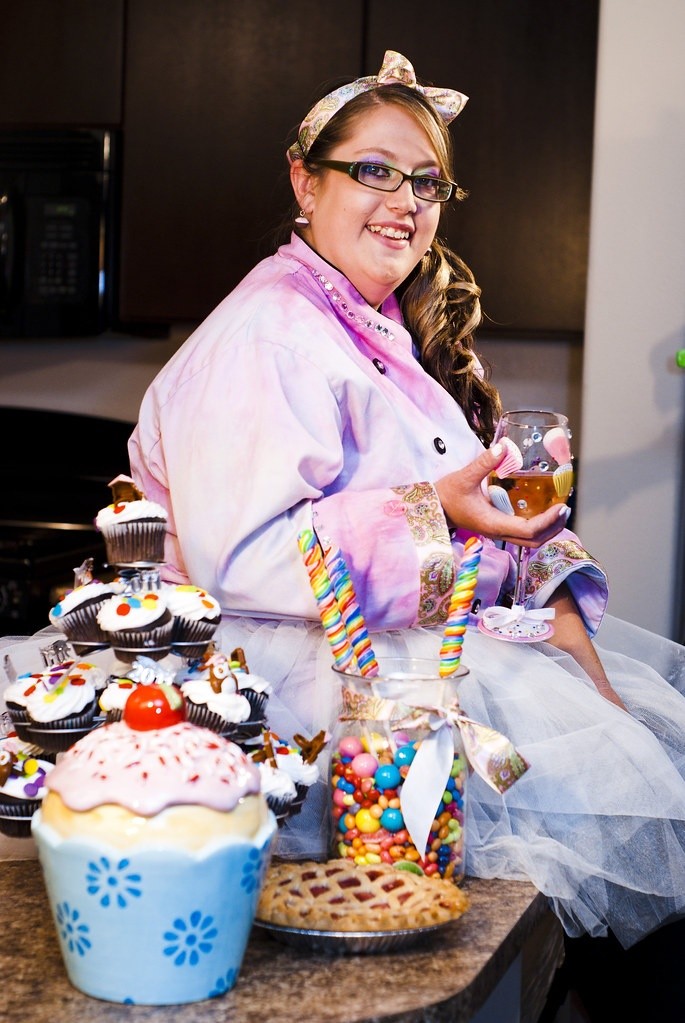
[31,810,277,1006]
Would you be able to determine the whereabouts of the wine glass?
[478,410,575,643]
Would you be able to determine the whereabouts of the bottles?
[325,655,469,885]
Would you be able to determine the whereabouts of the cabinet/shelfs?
[0,0,600,331]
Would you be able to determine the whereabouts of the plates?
[254,918,446,951]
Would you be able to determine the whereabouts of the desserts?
[0,475,484,933]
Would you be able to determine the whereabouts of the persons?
[127,49,685,1023]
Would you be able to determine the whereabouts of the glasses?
[314,155,458,203]
